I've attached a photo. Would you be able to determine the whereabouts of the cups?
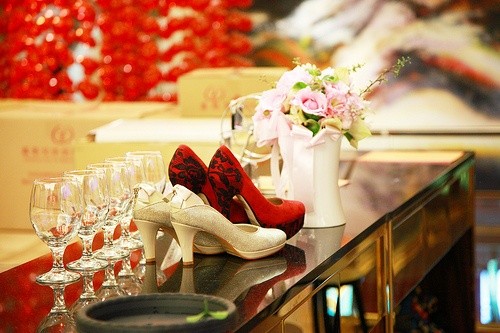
[127,151,167,198]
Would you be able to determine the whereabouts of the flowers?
[258,54,418,143]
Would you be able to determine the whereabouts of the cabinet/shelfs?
[0,148,478,333]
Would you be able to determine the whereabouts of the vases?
[280,128,346,230]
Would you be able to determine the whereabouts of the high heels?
[168,143,249,224]
[233,241,307,321]
[142,254,288,303]
[132,184,225,263]
[171,184,286,268]
[208,144,305,240]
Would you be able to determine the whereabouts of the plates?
[76,292,236,333]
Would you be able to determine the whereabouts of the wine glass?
[65,168,108,270]
[87,162,131,259]
[36,246,167,333]
[105,157,147,250]
[30,176,83,285]
